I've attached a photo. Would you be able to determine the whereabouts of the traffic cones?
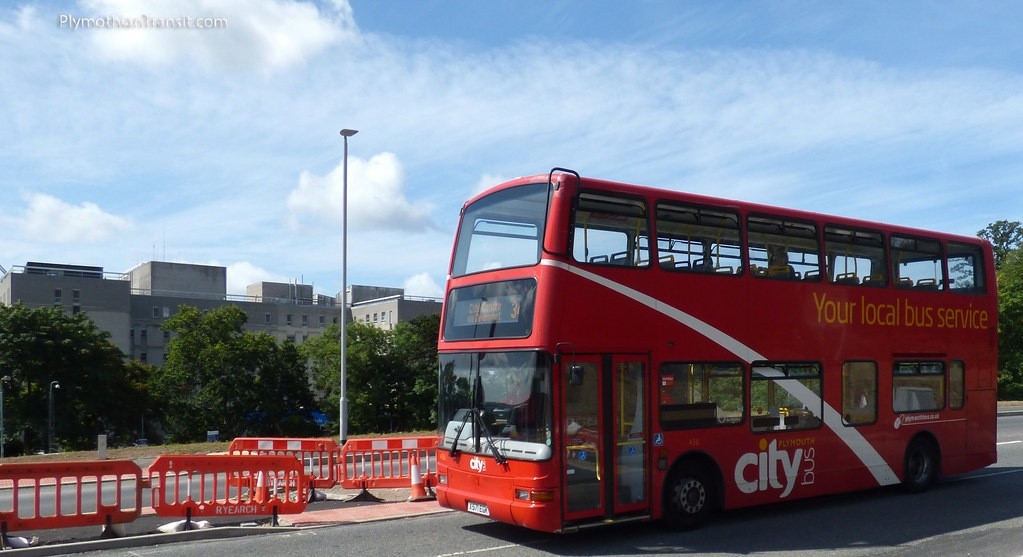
[410,453,436,503]
[252,469,269,506]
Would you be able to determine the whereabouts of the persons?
[758,247,795,280]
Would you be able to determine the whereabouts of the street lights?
[336,126,360,444]
[140,409,154,439]
[48,380,61,456]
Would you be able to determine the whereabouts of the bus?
[436,167,1001,534]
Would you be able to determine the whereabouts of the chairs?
[589,250,937,290]
